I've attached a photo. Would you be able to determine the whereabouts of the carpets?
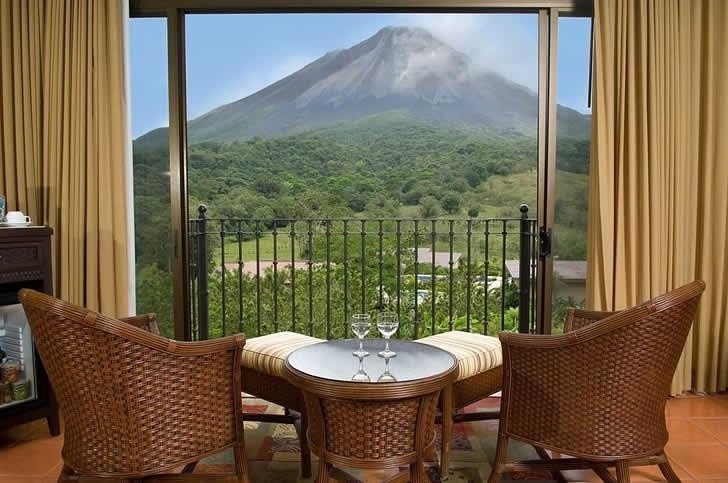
[145,396,552,483]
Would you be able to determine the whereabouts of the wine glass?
[377,356,401,382]
[350,355,371,382]
[349,313,372,357]
[375,310,400,358]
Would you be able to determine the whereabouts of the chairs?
[18,289,250,483]
[488,281,705,483]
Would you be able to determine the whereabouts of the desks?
[0,223,61,437]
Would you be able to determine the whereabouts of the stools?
[235,332,328,478]
[413,331,504,479]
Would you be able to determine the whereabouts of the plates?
[0,222,32,227]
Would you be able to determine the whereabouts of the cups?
[4,210,31,223]
